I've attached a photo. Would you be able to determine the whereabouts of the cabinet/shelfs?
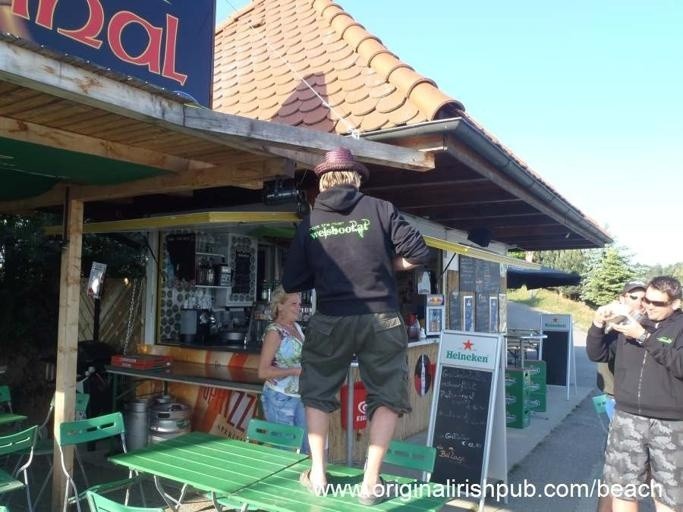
[194,251,233,289]
[242,300,312,349]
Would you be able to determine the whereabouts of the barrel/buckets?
[147,402,193,447]
[123,397,147,454]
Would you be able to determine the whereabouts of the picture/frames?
[425,305,444,337]
[488,296,500,332]
[463,295,475,332]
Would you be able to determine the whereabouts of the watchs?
[639,329,648,345]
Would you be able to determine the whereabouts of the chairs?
[0,384,437,512]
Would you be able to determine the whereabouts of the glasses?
[644,297,672,307]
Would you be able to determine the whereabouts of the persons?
[597,280,648,512]
[258,284,309,455]
[283,147,430,506]
[587,276,683,512]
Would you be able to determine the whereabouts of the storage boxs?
[505,360,547,429]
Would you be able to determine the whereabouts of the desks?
[106,429,459,512]
[505,328,549,369]
[104,360,267,457]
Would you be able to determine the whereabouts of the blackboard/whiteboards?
[421,329,501,498]
[538,314,570,388]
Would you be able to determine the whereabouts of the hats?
[622,280,647,296]
[312,147,371,183]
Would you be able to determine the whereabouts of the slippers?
[299,468,329,496]
[358,477,402,506]
